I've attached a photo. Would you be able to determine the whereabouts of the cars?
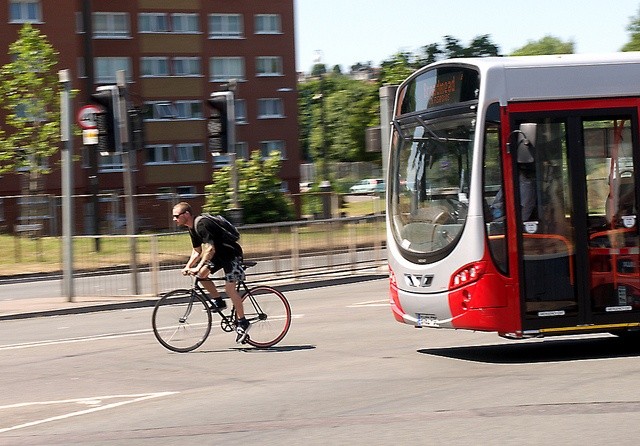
[349,177,385,196]
[318,179,332,191]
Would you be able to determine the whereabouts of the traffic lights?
[89,89,117,152]
[204,94,228,153]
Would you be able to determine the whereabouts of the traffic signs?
[76,103,105,129]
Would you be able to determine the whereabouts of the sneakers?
[202,301,227,312]
[236,320,252,344]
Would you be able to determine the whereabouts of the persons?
[481,163,536,219]
[171,202,253,343]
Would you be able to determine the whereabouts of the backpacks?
[194,212,241,249]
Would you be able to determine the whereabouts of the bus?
[383,47,640,347]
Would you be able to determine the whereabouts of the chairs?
[605,170,635,222]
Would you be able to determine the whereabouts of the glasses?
[172,212,186,219]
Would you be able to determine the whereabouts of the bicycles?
[152,261,291,352]
